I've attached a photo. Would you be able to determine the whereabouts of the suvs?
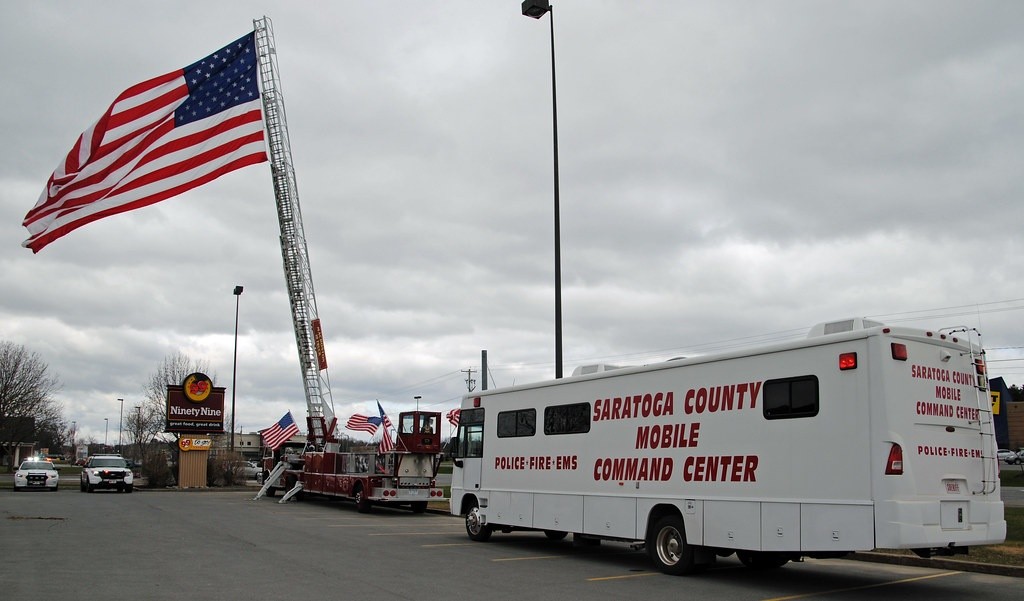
[80,453,134,494]
[1004,451,1024,465]
[230,460,264,479]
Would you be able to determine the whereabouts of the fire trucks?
[252,15,447,515]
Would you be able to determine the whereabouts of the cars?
[997,449,1015,462]
[75,458,87,465]
[13,461,62,492]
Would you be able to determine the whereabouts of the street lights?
[104,418,108,454]
[118,398,124,455]
[134,406,142,463]
[230,285,244,471]
[414,395,422,412]
[521,0,564,378]
[72,421,76,444]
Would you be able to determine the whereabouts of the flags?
[22,29,269,253]
[345,413,382,436]
[445,407,460,427]
[262,411,299,452]
[377,401,395,456]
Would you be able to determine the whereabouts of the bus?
[448,314,1008,576]
